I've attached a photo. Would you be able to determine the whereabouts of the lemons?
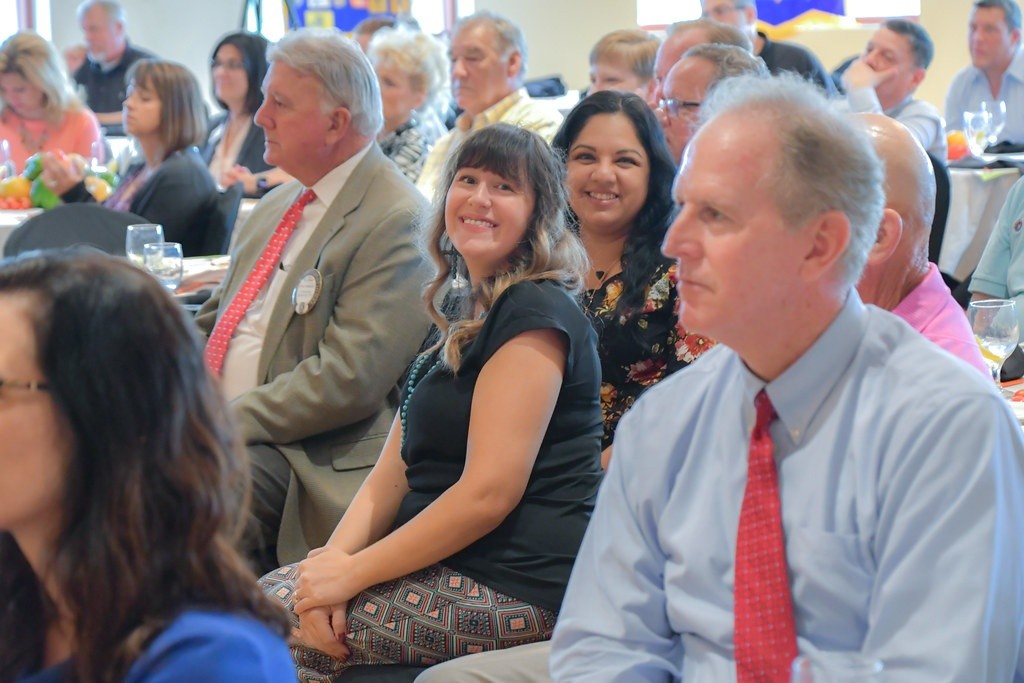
[974,335,1002,363]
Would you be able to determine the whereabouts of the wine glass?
[963,112,992,157]
[981,100,1007,147]
[970,301,1019,401]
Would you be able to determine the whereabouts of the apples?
[0,151,119,209]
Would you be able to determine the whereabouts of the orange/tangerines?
[946,130,969,160]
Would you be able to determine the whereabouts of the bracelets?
[257,177,267,188]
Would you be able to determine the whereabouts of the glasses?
[208,57,247,76]
[659,98,705,118]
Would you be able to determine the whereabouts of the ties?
[202,189,317,380]
[734,389,799,683]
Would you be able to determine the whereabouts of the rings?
[295,592,298,599]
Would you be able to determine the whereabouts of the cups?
[144,242,183,290]
[126,223,165,269]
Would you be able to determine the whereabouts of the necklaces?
[591,259,621,282]
[400,355,441,448]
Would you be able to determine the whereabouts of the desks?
[937,153,1024,283]
[137,255,231,308]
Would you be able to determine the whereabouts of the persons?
[701,0,837,97]
[194,14,442,568]
[841,20,948,164]
[415,77,1024,683]
[0,30,98,168]
[0,243,299,683]
[44,58,229,258]
[967,175,1024,345]
[554,91,719,467]
[943,0,1024,143]
[253,122,603,681]
[857,113,990,372]
[585,19,771,162]
[412,9,564,202]
[59,0,157,126]
[202,33,296,198]
